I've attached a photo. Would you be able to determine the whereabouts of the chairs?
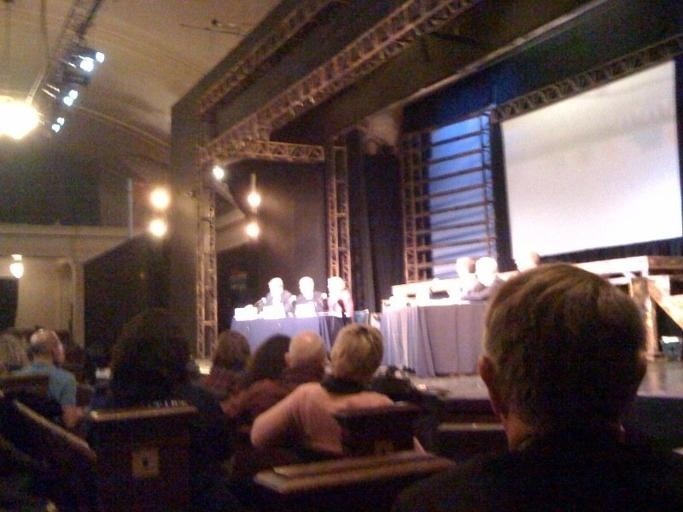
[353,309,369,326]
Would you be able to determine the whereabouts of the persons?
[462,256,506,301]
[382,262,683,510]
[325,277,353,314]
[254,276,294,314]
[285,276,326,311]
[450,254,476,298]
[0,306,425,510]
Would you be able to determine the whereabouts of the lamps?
[25,39,105,135]
